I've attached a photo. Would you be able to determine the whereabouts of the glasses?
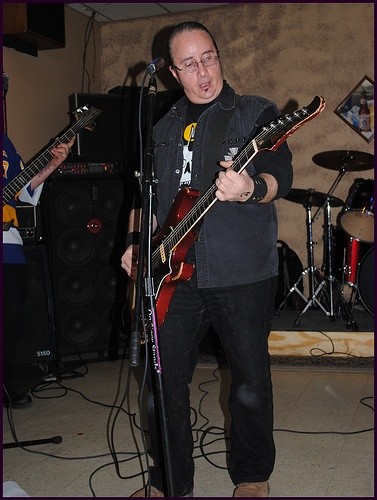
[171,51,217,74]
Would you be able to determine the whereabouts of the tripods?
[274,162,360,332]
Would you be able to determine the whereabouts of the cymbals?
[282,188,345,208]
[311,150,375,172]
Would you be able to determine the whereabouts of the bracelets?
[125,231,140,249]
[238,176,268,204]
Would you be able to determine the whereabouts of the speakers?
[34,169,143,364]
[2,3,66,51]
[69,92,149,164]
[0,242,57,372]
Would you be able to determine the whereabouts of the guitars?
[2,103,109,208]
[125,93,325,338]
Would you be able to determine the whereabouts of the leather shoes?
[3,387,33,410]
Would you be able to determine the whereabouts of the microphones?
[146,54,171,73]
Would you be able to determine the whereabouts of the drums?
[355,244,377,319]
[336,177,376,244]
[322,224,363,281]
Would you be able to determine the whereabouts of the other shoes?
[231,477,271,497]
[129,481,194,498]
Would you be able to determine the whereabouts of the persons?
[121,22,294,496]
[3,134,76,410]
